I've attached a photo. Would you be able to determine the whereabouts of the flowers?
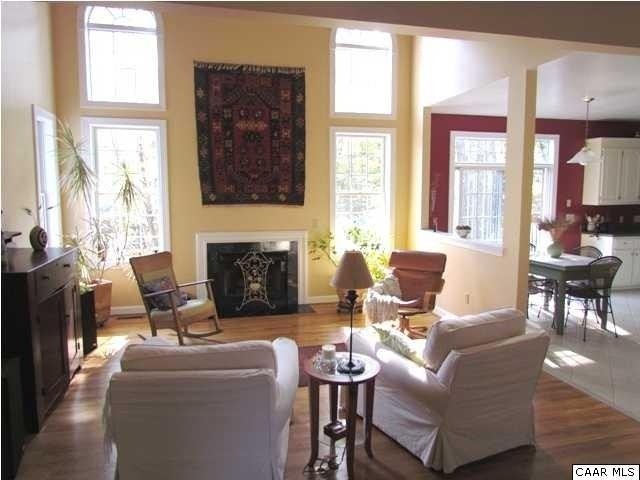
[532,212,580,243]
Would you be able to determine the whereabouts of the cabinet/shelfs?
[577,232,640,292]
[1,244,84,441]
[580,135,640,208]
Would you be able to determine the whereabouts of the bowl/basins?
[455,229,471,240]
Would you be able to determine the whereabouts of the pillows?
[371,321,431,368]
[139,275,188,312]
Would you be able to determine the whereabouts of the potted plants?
[61,233,99,358]
[306,222,393,316]
[46,115,141,329]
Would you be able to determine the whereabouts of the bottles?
[432,217,437,232]
[587,223,595,232]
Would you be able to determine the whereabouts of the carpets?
[525,284,640,428]
[293,340,350,391]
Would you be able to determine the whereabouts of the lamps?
[326,249,377,375]
[563,94,608,168]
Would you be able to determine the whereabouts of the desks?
[300,348,384,479]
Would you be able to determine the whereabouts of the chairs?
[363,248,450,341]
[338,302,554,477]
[127,249,224,349]
[102,333,302,479]
[521,241,627,344]
